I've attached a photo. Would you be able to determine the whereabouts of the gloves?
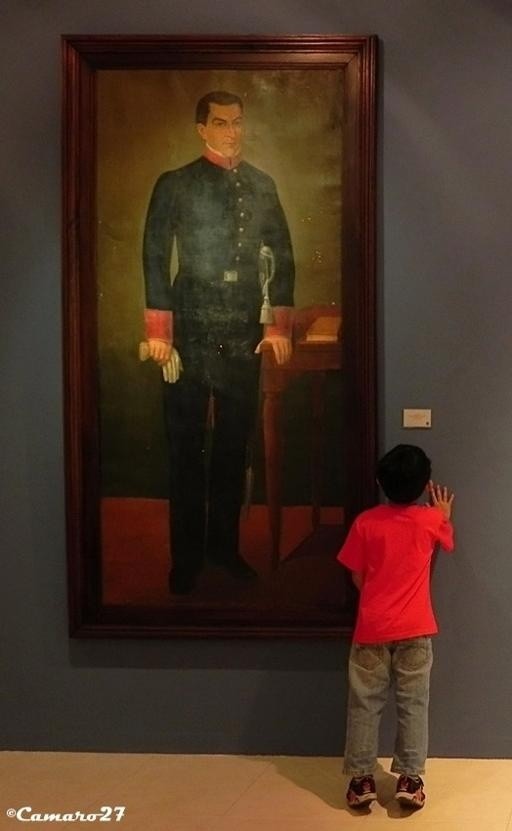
[136,340,186,385]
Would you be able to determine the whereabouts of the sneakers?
[394,773,426,807]
[204,545,260,582]
[345,775,379,810]
[169,556,203,598]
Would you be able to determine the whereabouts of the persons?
[139,87,297,596]
[337,440,454,808]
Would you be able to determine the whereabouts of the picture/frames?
[56,30,382,645]
